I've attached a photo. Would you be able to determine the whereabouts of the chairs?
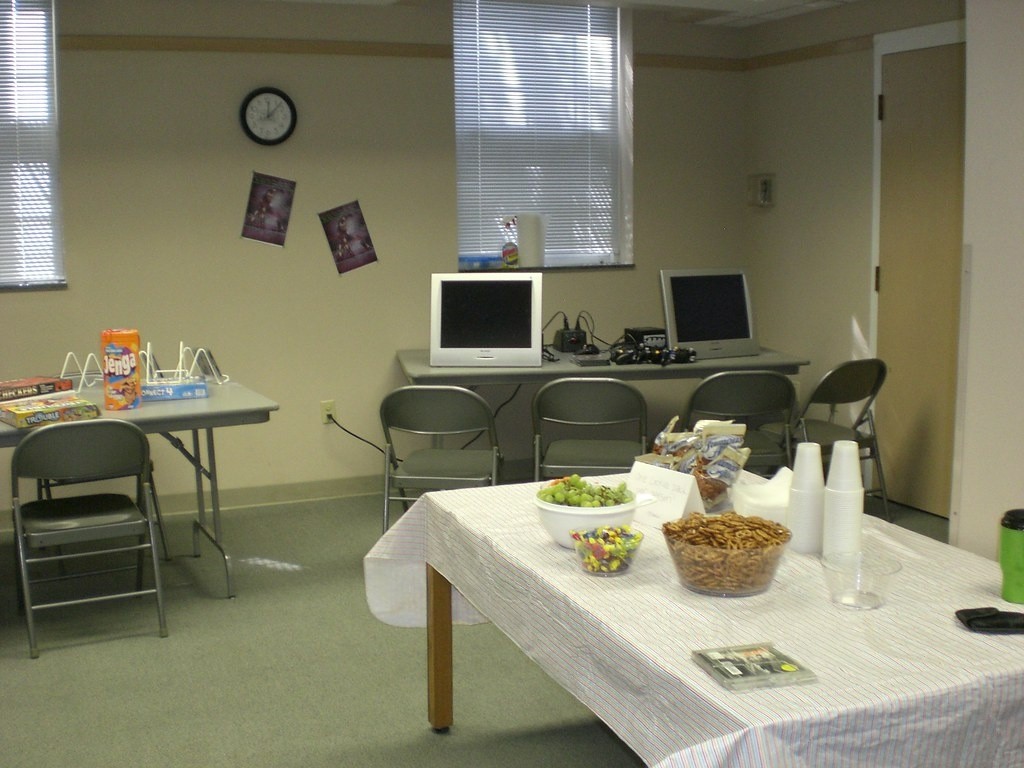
[681,367,798,480]
[377,383,505,544]
[527,373,651,482]
[9,418,174,660]
[38,367,172,571]
[761,359,890,522]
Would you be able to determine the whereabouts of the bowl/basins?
[821,551,902,610]
[662,515,793,598]
[570,528,645,577]
[533,482,656,549]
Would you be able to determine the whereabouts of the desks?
[0,372,280,602]
[420,473,1024,768]
[395,346,812,453]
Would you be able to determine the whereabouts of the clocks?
[240,85,299,147]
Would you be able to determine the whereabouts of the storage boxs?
[141,375,210,401]
[0,376,74,401]
[0,396,103,429]
[457,255,503,270]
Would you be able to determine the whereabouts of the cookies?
[662,513,793,593]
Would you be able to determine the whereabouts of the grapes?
[536,474,629,508]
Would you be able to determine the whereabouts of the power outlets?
[321,400,336,424]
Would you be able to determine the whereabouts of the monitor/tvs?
[430,273,543,368]
[659,269,762,360]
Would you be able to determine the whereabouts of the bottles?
[999,509,1024,605]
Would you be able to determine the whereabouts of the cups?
[823,440,865,557]
[788,442,824,553]
